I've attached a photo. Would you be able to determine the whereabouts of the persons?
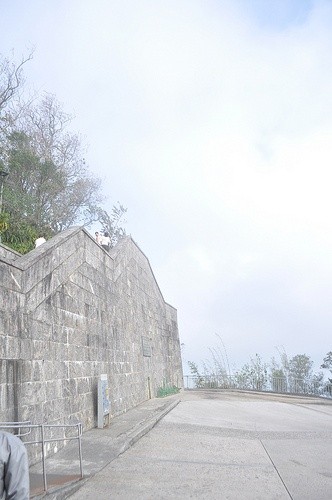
[34,232,46,247]
[100,232,110,250]
[0,430,30,500]
[95,231,102,246]
